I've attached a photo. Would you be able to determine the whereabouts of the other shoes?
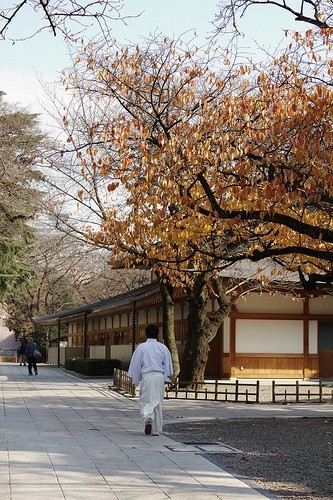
[144,419,153,435]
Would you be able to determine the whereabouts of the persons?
[24,337,41,377]
[16,343,27,367]
[126,322,175,438]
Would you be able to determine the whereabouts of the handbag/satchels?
[33,342,41,361]
